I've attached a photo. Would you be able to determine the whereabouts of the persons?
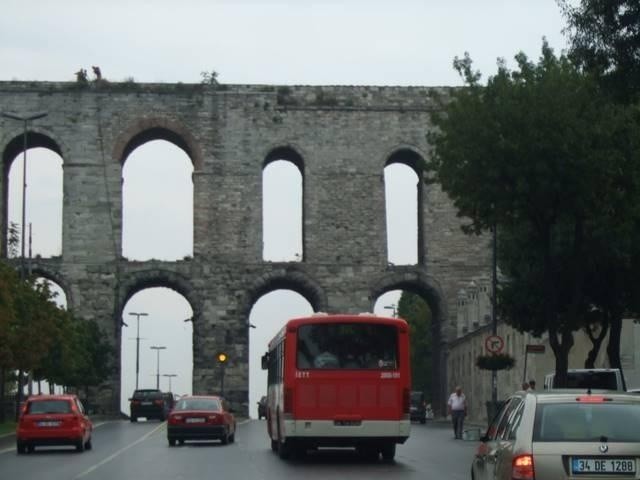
[314,342,341,368]
[529,381,536,390]
[447,386,470,440]
[523,383,529,391]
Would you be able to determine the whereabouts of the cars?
[16,394,94,454]
[257,396,267,420]
[167,395,235,447]
[470,368,640,480]
[130,389,174,422]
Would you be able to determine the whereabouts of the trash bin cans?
[486,401,500,426]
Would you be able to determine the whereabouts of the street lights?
[0,112,49,419]
[128,311,148,389]
[150,346,177,391]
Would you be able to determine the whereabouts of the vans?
[412,392,426,424]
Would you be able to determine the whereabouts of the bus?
[262,315,412,459]
